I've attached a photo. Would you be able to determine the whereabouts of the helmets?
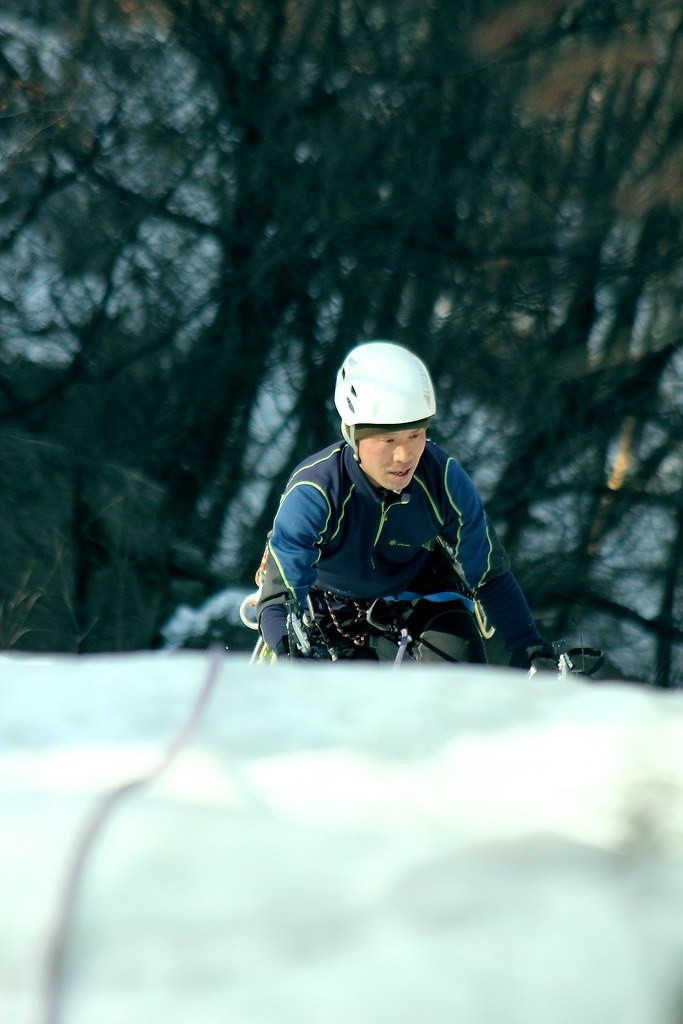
[334,341,437,430]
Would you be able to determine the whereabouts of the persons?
[256,341,559,672]
